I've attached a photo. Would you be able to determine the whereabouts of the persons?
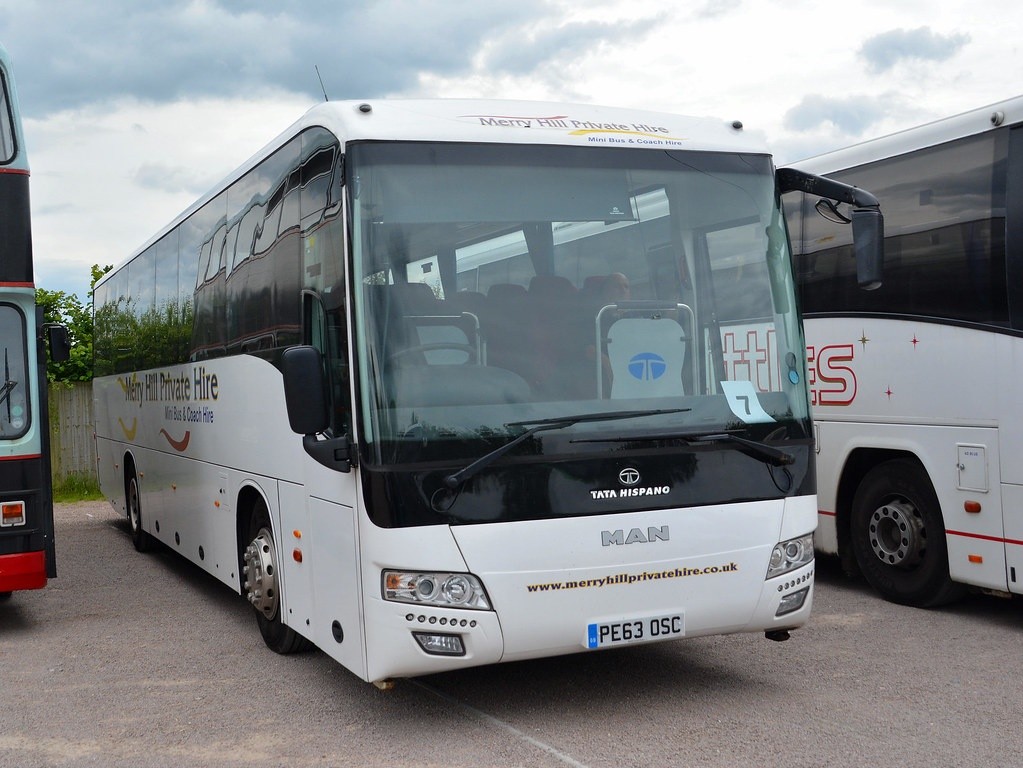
[579,273,645,385]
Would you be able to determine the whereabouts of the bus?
[83,62,889,691]
[772,94,1023,613]
[1,45,58,607]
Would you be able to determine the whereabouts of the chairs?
[325,269,660,407]
